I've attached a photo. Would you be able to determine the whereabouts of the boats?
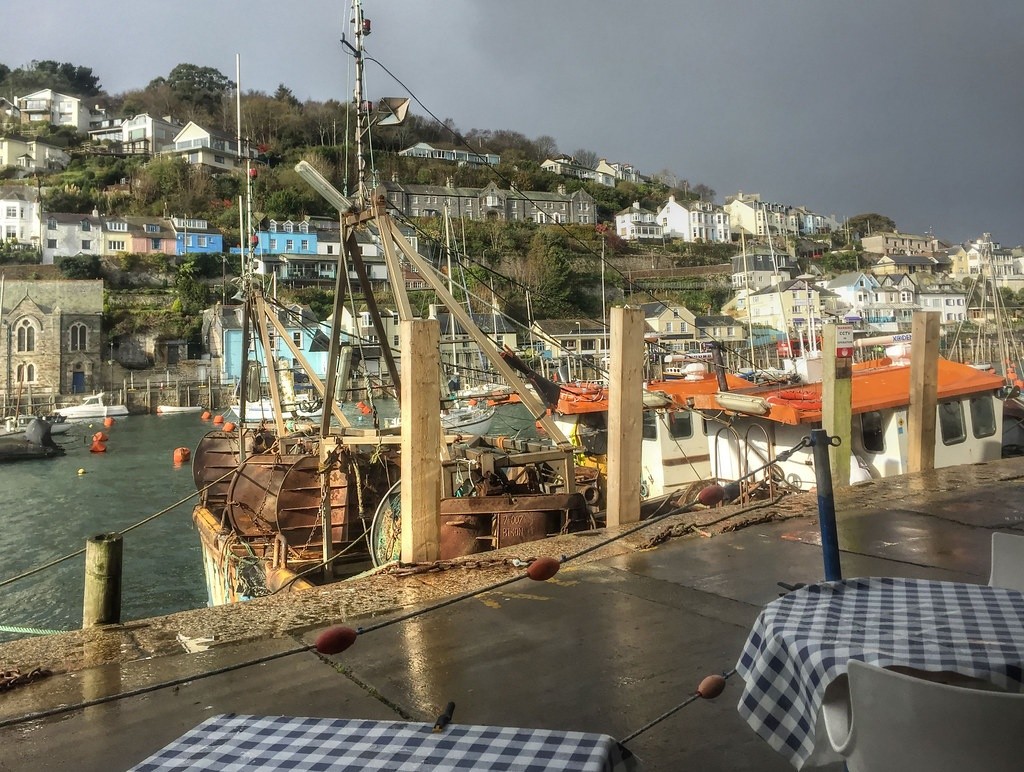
[7,415,72,435]
[158,405,201,414]
[53,393,130,418]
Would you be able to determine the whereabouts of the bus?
[777,337,821,356]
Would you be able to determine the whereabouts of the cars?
[601,355,610,364]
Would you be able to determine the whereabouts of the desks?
[735,575,1024,771]
[124,711,641,772]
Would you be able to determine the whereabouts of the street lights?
[575,322,581,355]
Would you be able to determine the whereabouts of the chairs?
[987,532,1024,594]
[816,657,1023,771]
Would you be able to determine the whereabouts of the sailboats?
[190,50,1024,600]
